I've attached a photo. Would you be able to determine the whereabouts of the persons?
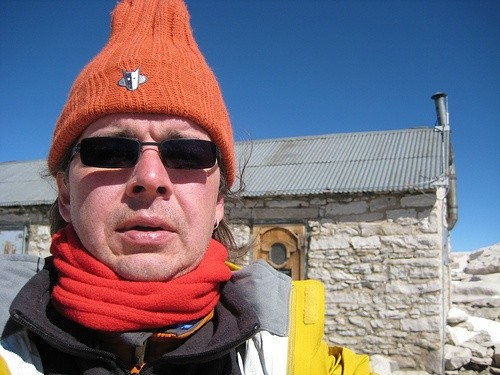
[0,0,386,375]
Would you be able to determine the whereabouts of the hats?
[48,0,236,192]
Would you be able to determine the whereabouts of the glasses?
[70,136,220,170]
